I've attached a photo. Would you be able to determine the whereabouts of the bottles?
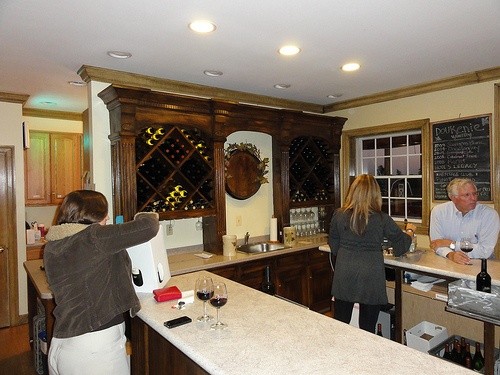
[459,337,466,352]
[132,123,216,214]
[472,343,484,371]
[451,339,459,362]
[476,258,491,294]
[288,136,334,202]
[375,323,383,337]
[462,343,474,371]
[442,343,451,361]
[262,266,275,294]
[453,344,464,367]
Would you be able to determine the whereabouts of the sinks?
[236,243,293,254]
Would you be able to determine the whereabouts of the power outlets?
[166,225,173,235]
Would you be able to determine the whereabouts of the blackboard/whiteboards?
[430,113,496,204]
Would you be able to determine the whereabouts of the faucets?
[238,232,254,247]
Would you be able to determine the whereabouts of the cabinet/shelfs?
[23,232,335,375]
[23,129,84,207]
[318,233,500,358]
[124,271,483,375]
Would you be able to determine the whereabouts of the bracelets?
[405,229,414,233]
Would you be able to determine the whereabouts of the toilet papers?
[269,218,277,241]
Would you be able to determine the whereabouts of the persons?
[46,190,160,375]
[329,174,416,336]
[430,177,500,266]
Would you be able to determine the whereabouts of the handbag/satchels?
[125,212,171,292]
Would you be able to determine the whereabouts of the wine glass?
[210,281,229,329]
[289,203,335,237]
[195,277,214,322]
[460,239,474,265]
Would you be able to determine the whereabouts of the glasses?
[454,191,479,198]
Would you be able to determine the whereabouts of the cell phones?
[164,316,192,328]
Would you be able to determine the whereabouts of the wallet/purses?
[153,286,182,302]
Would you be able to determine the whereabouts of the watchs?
[450,241,456,250]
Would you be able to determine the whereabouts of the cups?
[283,227,297,246]
[222,235,237,256]
[382,229,417,255]
[38,224,45,238]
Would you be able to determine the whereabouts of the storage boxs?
[427,335,500,375]
